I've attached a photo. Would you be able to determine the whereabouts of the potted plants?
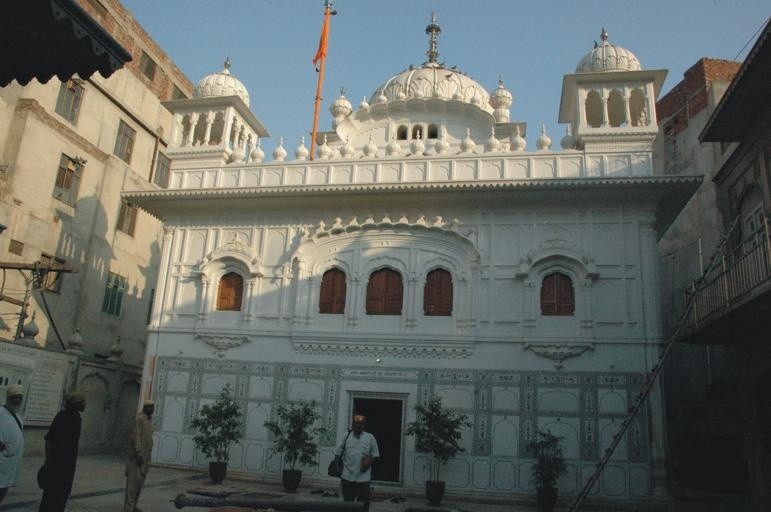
[524,426,569,510]
[404,391,473,505]
[262,398,333,492]
[189,382,245,484]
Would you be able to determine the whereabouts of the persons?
[333,414,379,510]
[0,383,27,502]
[120,399,154,512]
[36,390,87,512]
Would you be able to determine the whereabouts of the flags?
[312,8,330,66]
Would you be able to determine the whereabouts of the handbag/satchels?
[328,459,344,477]
